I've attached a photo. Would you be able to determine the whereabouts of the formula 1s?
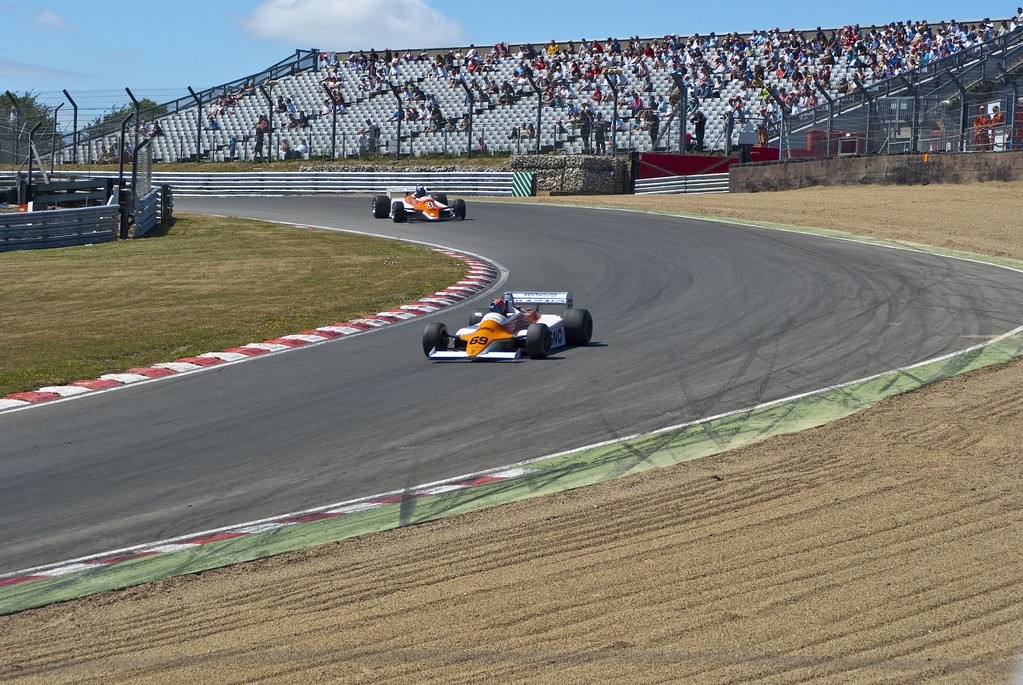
[422,290,593,363]
[373,184,467,224]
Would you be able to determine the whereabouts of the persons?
[317,23,898,123]
[81,79,772,165]
[1006,98,1023,151]
[489,299,510,318]
[414,186,427,199]
[894,7,1023,84]
[972,106,992,151]
[987,105,1004,150]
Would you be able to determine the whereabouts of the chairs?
[54,30,1005,160]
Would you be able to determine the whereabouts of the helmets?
[416,188,425,197]
[489,298,508,315]
